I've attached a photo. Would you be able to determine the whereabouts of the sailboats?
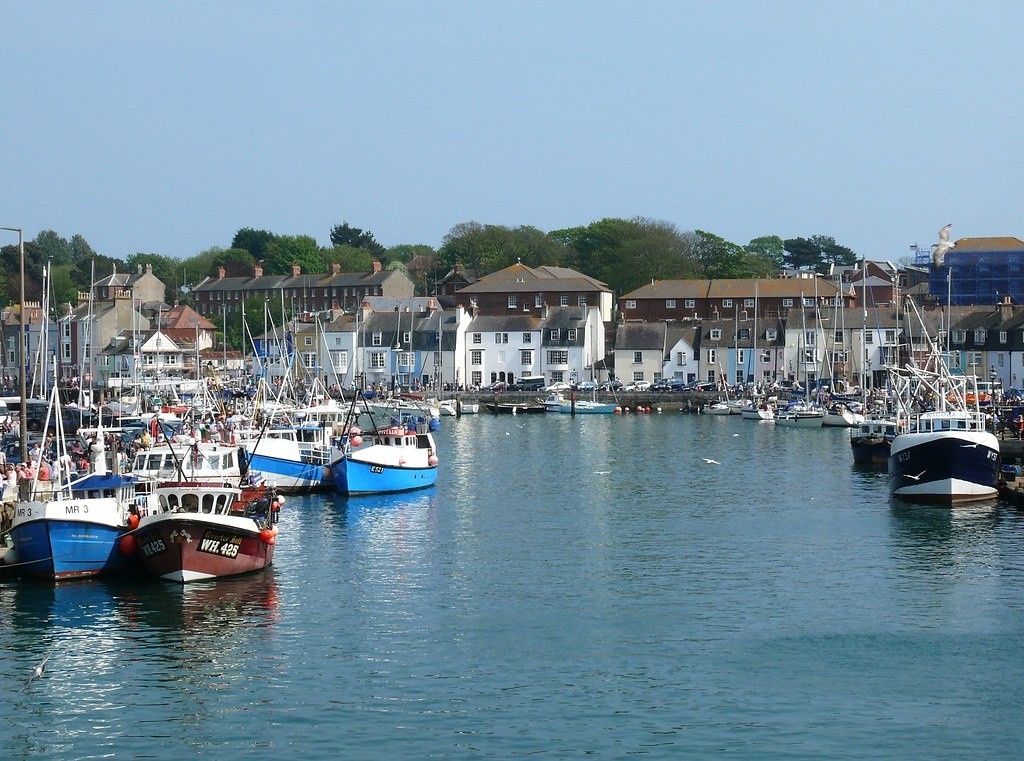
[0,258,1023,590]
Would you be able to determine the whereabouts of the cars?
[569,381,598,392]
[479,381,510,392]
[680,381,715,391]
[589,381,623,392]
[539,381,571,392]
[618,381,650,392]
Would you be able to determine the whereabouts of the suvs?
[505,376,544,392]
[649,378,685,392]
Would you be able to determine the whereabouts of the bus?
[975,381,1004,395]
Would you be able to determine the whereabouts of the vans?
[808,378,842,392]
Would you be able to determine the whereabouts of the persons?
[182,410,235,443]
[77,432,127,474]
[446,381,472,391]
[29,444,40,461]
[367,383,371,391]
[0,457,60,503]
[2,417,8,438]
[134,434,142,447]
[1014,415,1024,440]
[757,380,762,394]
[680,384,685,392]
[351,385,354,390]
[72,441,84,461]
[329,384,338,394]
[142,429,150,447]
[0,446,6,474]
[718,380,722,392]
[88,436,96,459]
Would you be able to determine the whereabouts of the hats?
[21,462,27,467]
